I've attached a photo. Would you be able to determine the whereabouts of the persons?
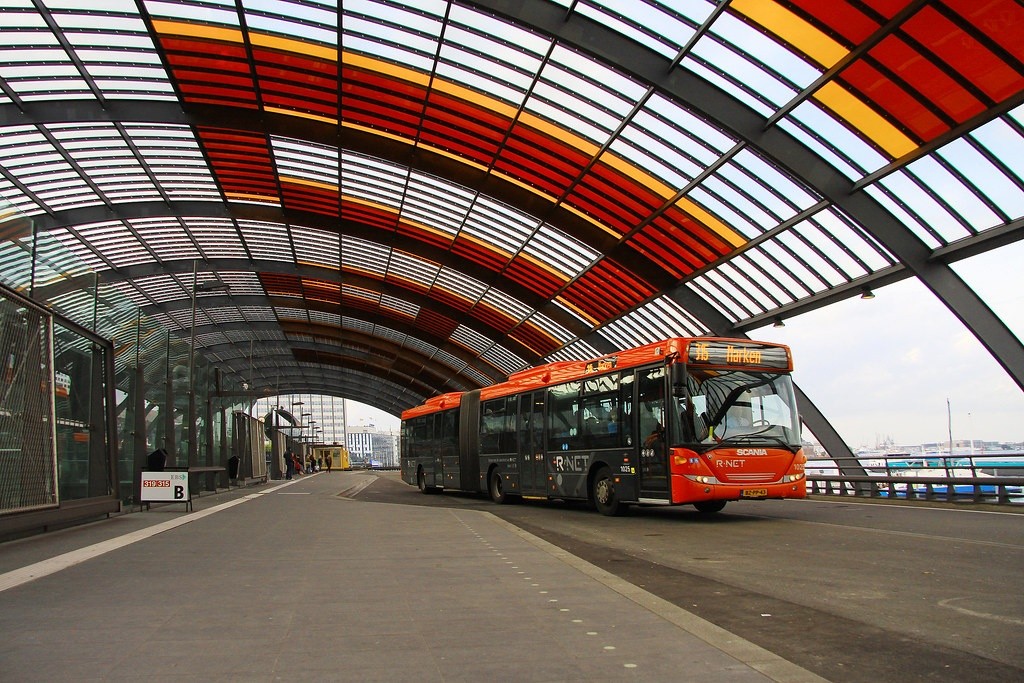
[727,406,750,428]
[292,453,317,475]
[607,408,618,433]
[324,455,332,473]
[681,403,700,440]
[283,447,295,480]
[318,457,323,471]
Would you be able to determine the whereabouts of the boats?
[867,460,888,473]
[866,399,1022,499]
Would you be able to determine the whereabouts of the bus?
[401,336,807,518]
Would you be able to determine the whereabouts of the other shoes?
[290,478,295,480]
